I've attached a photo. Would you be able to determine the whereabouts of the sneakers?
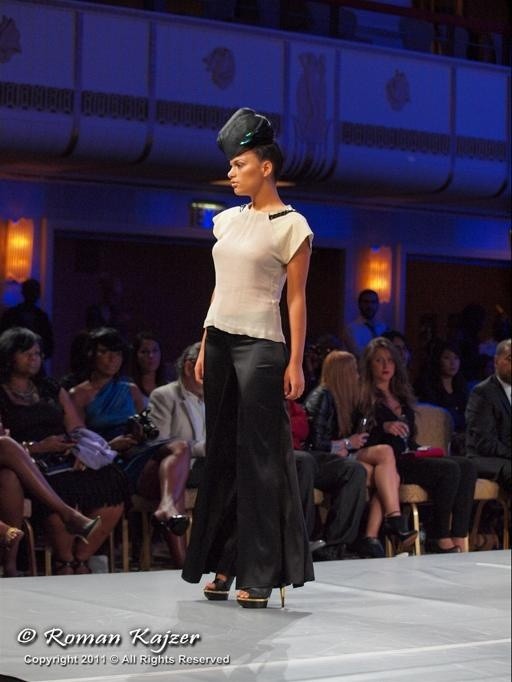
[428,545,462,554]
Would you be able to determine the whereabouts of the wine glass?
[397,426,414,456]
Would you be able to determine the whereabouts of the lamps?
[365,243,395,306]
[0,212,38,288]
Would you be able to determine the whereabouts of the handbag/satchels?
[415,446,447,462]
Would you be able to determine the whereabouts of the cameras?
[128,407,159,440]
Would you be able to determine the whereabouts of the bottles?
[357,417,370,447]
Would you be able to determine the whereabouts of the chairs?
[0,394,511,583]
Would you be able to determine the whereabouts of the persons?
[450,304,484,351]
[340,288,391,353]
[412,311,446,365]
[86,278,150,342]
[1,277,58,361]
[479,316,511,360]
[180,138,318,612]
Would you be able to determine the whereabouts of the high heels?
[383,512,420,555]
[151,513,191,538]
[63,515,102,546]
[0,526,25,552]
[234,585,287,609]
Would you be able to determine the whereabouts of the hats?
[215,106,276,161]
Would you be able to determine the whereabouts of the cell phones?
[61,438,78,444]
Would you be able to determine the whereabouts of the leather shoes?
[363,537,385,558]
[307,538,326,552]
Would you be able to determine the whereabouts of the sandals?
[54,559,92,576]
[203,573,235,602]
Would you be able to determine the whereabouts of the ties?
[365,322,378,339]
